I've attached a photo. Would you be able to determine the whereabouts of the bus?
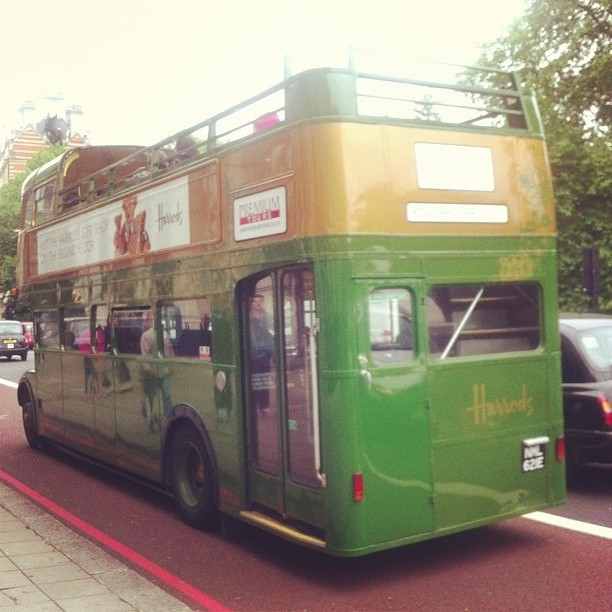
[19,66,565,560]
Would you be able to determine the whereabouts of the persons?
[243,292,276,428]
[163,136,202,167]
[141,309,177,431]
[125,148,167,185]
[79,329,102,395]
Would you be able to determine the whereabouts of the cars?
[563,314,612,482]
[21,322,34,346]
[0,320,28,363]
